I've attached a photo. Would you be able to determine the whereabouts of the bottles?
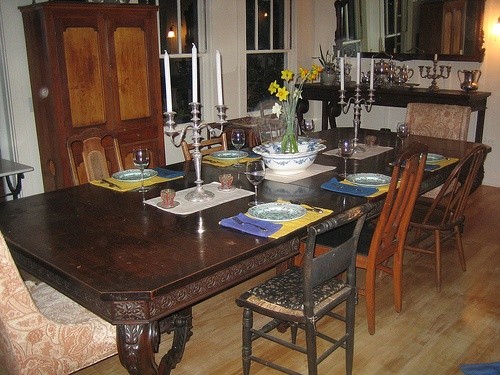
[457,68,482,94]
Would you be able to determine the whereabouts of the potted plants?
[314,44,336,86]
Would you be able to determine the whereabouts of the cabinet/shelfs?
[17,0,167,194]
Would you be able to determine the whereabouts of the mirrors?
[333,0,486,63]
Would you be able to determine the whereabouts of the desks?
[294,83,491,143]
[0,160,34,203]
[0,127,492,375]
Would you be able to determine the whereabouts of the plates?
[210,150,249,160]
[426,153,444,165]
[346,172,391,188]
[113,169,157,181]
[246,203,306,223]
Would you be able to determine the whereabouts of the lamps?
[167,24,177,38]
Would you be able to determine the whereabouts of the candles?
[338,50,340,57]
[345,54,347,65]
[163,48,173,113]
[435,54,437,60]
[370,57,373,88]
[215,49,224,106]
[340,58,344,89]
[191,43,197,103]
[357,52,360,82]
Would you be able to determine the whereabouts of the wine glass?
[231,128,245,168]
[246,159,266,206]
[300,118,315,137]
[397,121,409,149]
[337,138,355,179]
[133,146,150,193]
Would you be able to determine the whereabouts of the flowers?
[269,63,324,116]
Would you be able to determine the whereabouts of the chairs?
[66,128,125,185]
[379,143,487,292]
[205,116,284,148]
[235,202,376,375]
[180,132,228,161]
[292,145,428,336]
[406,102,472,142]
[0,232,119,375]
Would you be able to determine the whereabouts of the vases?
[282,113,298,153]
